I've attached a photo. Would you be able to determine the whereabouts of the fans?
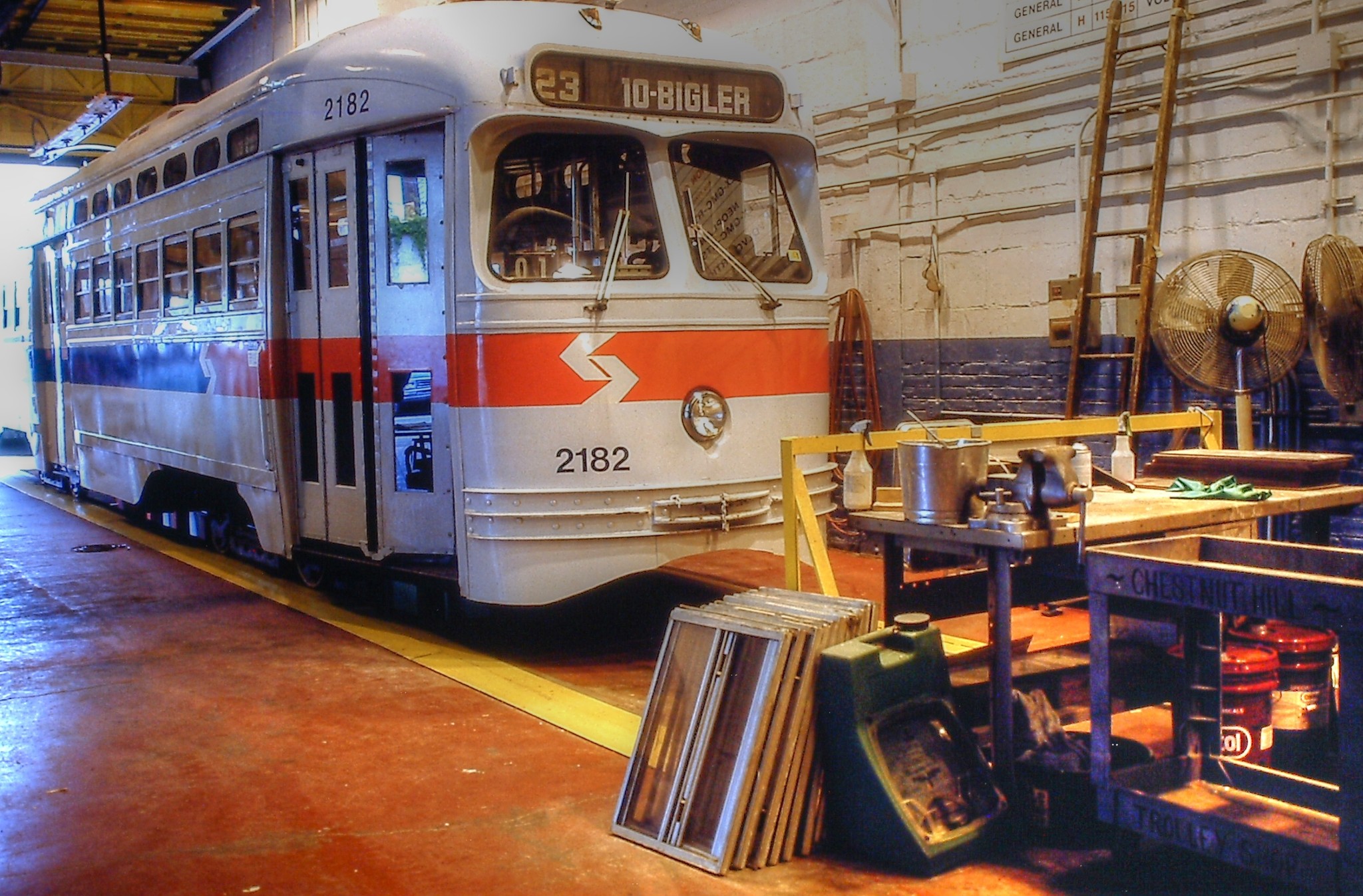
[1148,234,1363,450]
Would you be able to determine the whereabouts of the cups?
[1070,449,1093,489]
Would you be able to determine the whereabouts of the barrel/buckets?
[1228,618,1337,771]
[895,408,994,525]
[813,612,1011,879]
[1167,643,1282,768]
[1011,731,1155,852]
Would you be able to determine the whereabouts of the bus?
[24,0,833,605]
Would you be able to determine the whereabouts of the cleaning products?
[1110,410,1137,482]
[843,418,876,509]
